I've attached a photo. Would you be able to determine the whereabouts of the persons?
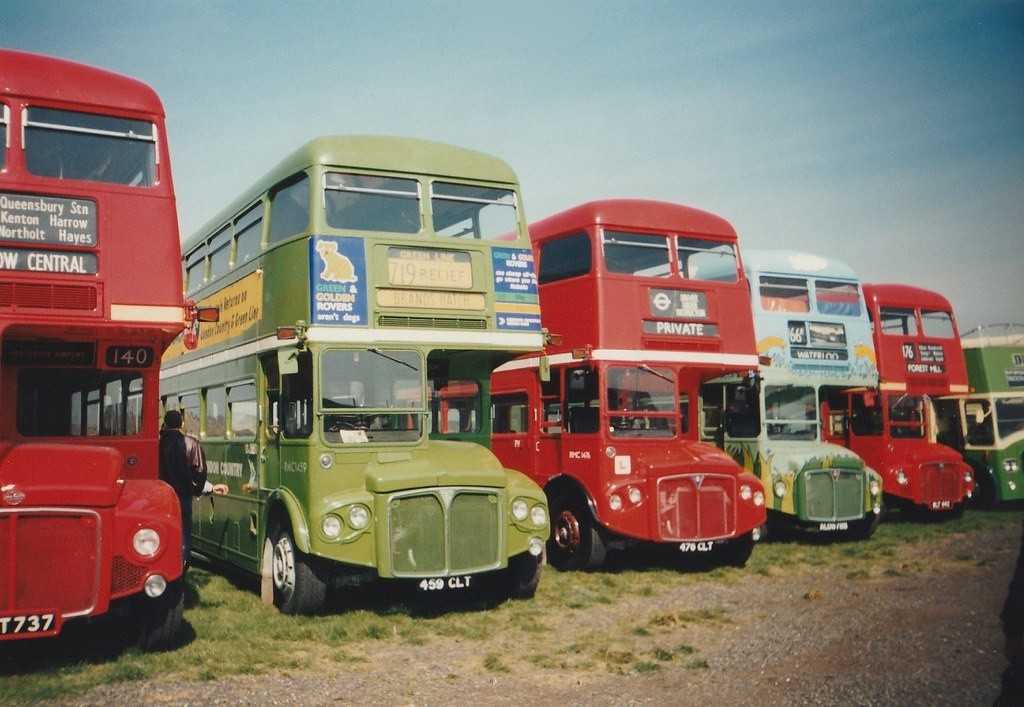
[159,409,229,575]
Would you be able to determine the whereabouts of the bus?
[431,250,883,542]
[406,198,772,575]
[726,283,973,522]
[96,135,563,616]
[889,322,1024,510]
[0,48,197,650]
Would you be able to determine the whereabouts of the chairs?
[570,407,599,434]
[970,423,993,445]
[852,413,882,435]
[630,405,670,430]
[727,412,753,436]
